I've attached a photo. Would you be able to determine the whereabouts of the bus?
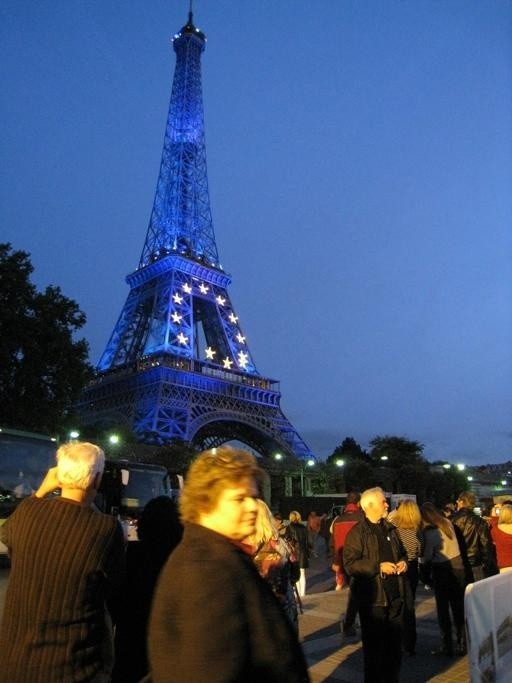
[0,427,77,569]
[91,459,184,548]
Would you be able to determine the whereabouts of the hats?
[344,504,360,512]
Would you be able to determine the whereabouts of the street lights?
[275,454,315,496]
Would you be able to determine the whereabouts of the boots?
[431,631,464,656]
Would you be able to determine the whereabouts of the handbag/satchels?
[420,563,432,586]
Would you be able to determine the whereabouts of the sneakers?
[341,625,357,635]
